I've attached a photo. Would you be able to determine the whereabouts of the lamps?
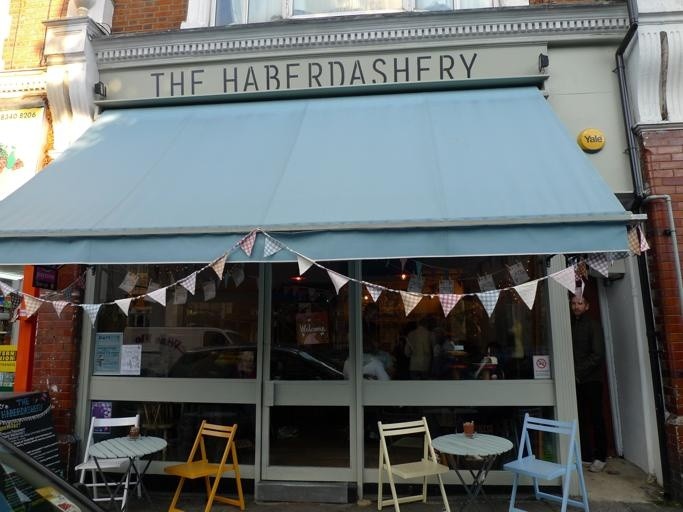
[538,53,550,74]
[94,80,107,98]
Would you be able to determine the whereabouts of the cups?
[463,423,475,439]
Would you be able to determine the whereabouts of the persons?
[570,286,610,474]
[343,298,504,440]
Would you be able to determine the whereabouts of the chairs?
[375,410,591,512]
[74,413,245,512]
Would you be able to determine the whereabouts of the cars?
[166,344,423,449]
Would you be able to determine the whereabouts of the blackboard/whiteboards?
[0,391,64,512]
